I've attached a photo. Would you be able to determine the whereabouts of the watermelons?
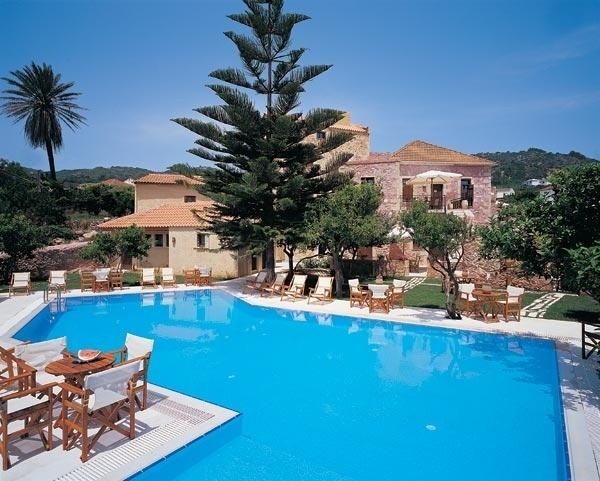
[78,350,100,361]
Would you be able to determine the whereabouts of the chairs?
[241,270,407,313]
[1,332,154,471]
[7,265,214,295]
[456,283,525,324]
